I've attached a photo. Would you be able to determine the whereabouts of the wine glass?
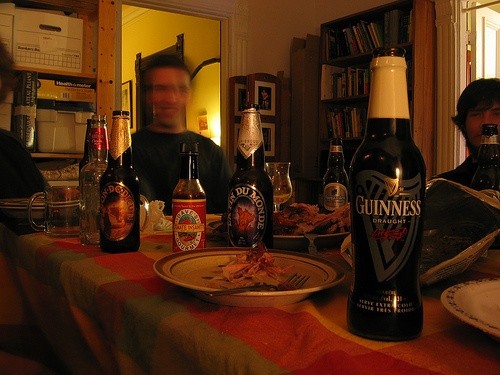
[263,162,292,212]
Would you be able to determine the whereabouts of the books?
[321,64,369,100]
[327,108,361,138]
[326,9,399,60]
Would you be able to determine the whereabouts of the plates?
[154,246,344,306]
[340,225,500,285]
[440,277,500,340]
[206,214,350,244]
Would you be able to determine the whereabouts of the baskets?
[340,217,500,286]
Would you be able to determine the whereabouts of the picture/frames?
[230,73,280,161]
[122,80,133,129]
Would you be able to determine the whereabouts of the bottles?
[78,119,93,187]
[99,110,140,253]
[171,142,207,255]
[225,103,274,251]
[321,136,349,214]
[84,113,110,245]
[469,123,500,252]
[349,45,425,342]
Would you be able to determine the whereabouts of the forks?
[205,272,310,297]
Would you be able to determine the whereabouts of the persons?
[131,53,233,215]
[0,39,46,200]
[427,78,500,251]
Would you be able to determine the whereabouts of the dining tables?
[0,214,500,375]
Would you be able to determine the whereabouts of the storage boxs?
[0,2,96,187]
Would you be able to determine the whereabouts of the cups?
[27,186,81,238]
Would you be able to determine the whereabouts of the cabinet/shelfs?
[291,0,435,195]
